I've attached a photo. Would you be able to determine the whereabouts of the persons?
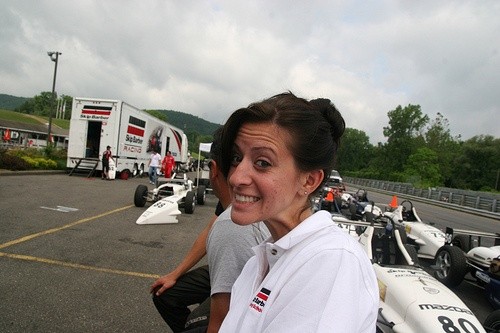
[219,92,379,333]
[148,149,161,185]
[206,125,272,333]
[102,146,115,180]
[149,200,225,333]
[162,151,176,178]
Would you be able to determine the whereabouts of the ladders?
[69,158,100,177]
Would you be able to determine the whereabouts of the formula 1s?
[135,170,205,224]
[316,187,499,333]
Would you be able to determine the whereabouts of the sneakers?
[150,180,155,184]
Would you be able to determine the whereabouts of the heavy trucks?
[67,97,188,177]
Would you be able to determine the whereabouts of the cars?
[325,170,342,183]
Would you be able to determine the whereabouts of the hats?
[107,146,111,148]
